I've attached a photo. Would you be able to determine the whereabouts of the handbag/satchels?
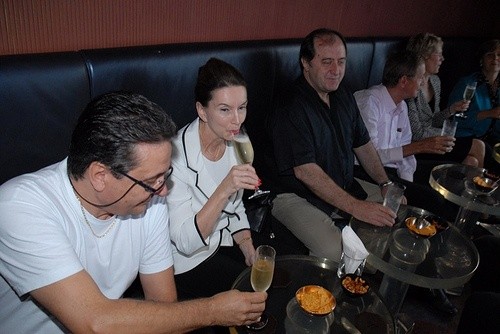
[244,189,277,233]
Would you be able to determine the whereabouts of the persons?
[405,32,487,173]
[1,90,268,334]
[350,40,461,318]
[447,35,500,172]
[164,56,262,334]
[256,27,407,320]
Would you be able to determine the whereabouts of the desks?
[225,256,398,334]
[343,203,480,316]
[406,164,500,317]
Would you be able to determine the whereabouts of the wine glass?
[245,245,276,329]
[454,80,477,118]
[230,126,270,200]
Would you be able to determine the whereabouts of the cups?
[440,119,458,153]
[383,186,405,215]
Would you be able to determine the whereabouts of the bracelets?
[380,180,393,188]
[448,105,454,118]
[234,236,251,246]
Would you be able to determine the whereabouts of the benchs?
[0,37,412,226]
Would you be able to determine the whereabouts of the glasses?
[110,165,173,195]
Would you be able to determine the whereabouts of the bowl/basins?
[426,215,449,234]
[472,176,498,194]
[404,216,437,239]
[295,285,337,318]
[340,273,370,297]
